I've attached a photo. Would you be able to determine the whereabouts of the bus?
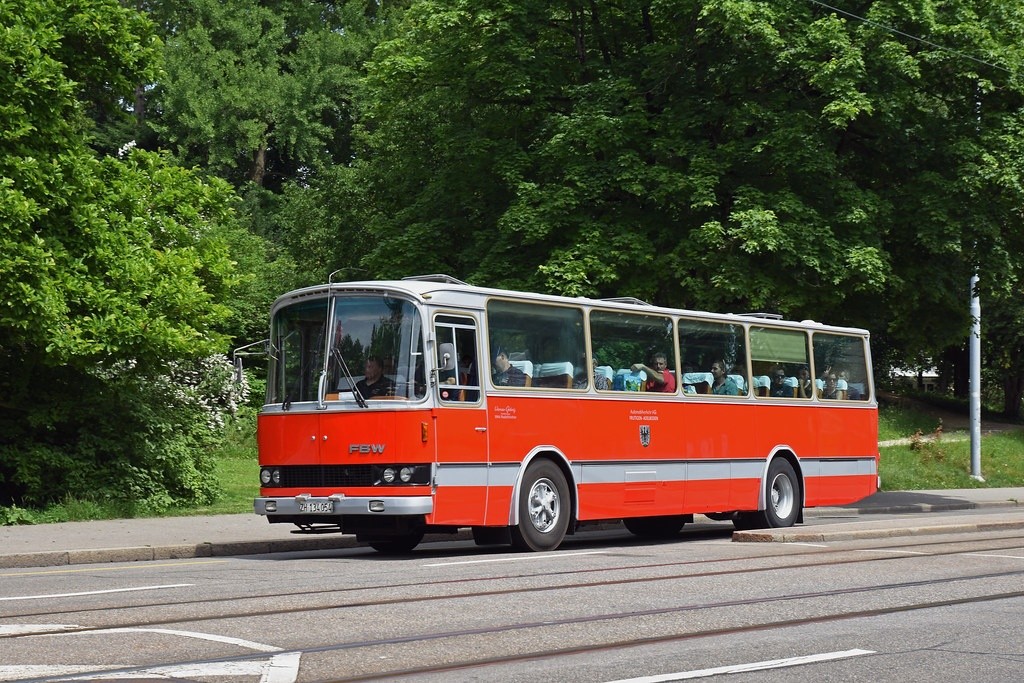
[232,270,881,557]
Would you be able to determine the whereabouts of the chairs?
[338,359,866,401]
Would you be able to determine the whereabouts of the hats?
[491,344,502,365]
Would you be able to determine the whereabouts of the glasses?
[828,378,837,382]
[773,374,785,379]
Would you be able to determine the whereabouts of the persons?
[710,360,739,396]
[768,365,794,398]
[492,347,525,387]
[573,353,611,390]
[797,366,861,400]
[630,353,675,393]
[352,355,394,396]
[740,363,760,396]
